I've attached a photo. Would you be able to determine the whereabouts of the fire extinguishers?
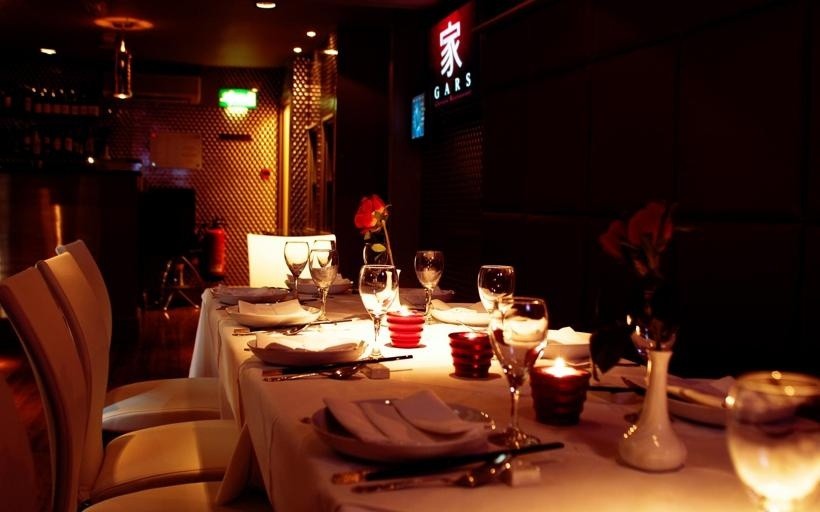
[206,218,229,277]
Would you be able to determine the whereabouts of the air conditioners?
[102,71,202,106]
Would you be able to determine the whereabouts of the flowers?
[354,194,397,268]
[596,201,678,352]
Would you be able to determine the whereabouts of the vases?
[617,353,689,471]
[378,270,402,328]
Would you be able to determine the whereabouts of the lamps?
[113,25,133,100]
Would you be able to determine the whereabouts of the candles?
[530,356,591,426]
[388,304,423,349]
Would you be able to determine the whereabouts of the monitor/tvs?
[409,92,427,141]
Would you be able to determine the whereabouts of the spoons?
[352,455,517,495]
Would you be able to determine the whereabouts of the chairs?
[35,250,240,511]
[246,233,338,287]
[55,238,231,433]
[0,264,263,512]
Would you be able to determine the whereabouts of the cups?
[387,311,424,347]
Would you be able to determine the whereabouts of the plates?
[225,301,323,328]
[286,276,352,293]
[622,377,733,419]
[544,326,593,361]
[246,330,369,368]
[308,397,493,451]
[212,282,286,303]
[429,299,494,327]
[397,283,454,300]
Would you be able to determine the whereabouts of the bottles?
[0,87,101,156]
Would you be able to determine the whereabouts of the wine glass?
[476,264,514,316]
[358,265,399,363]
[727,372,820,511]
[285,240,309,297]
[414,250,445,323]
[308,250,340,321]
[491,296,548,447]
